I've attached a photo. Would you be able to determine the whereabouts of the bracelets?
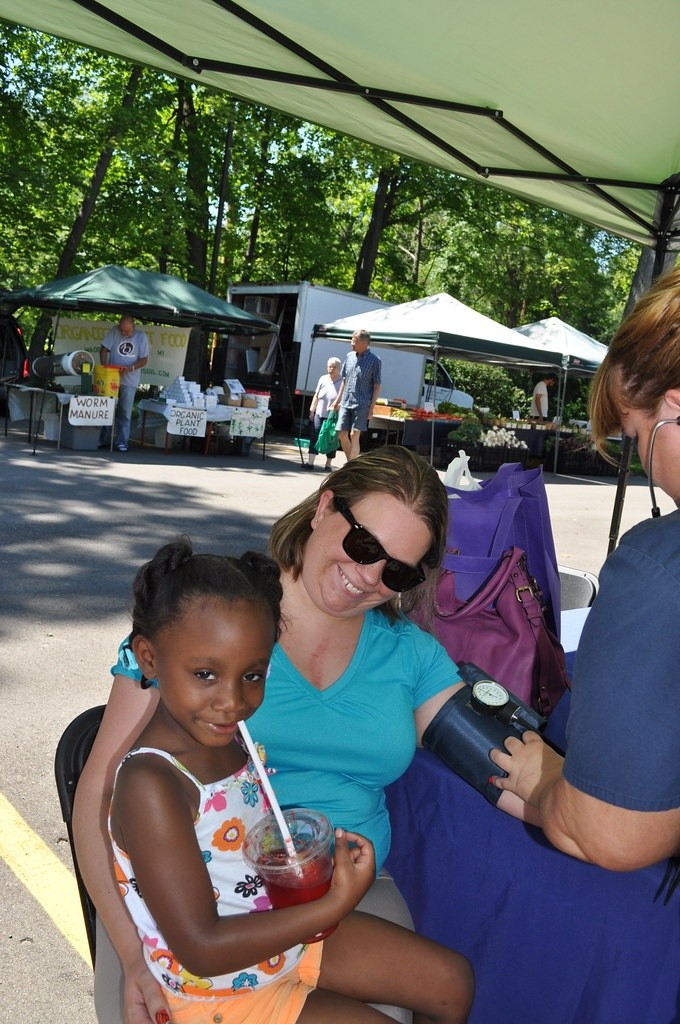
[132,364,134,371]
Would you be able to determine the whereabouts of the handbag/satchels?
[434,462,561,641]
[313,409,340,454]
[400,544,569,721]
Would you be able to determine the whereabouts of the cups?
[64,384,74,394]
[242,808,339,944]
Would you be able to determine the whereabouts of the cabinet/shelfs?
[136,398,272,461]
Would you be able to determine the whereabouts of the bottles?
[81,363,91,395]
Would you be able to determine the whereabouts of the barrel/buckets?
[256,391,272,409]
[93,364,128,397]
[242,390,256,400]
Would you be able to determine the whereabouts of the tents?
[299,291,609,474]
[0,265,304,464]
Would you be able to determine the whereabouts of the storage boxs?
[190,433,252,456]
[155,428,175,449]
[43,413,61,441]
[159,376,257,408]
[59,417,103,451]
[440,439,530,471]
[372,405,399,417]
[541,448,622,475]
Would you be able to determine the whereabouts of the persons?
[301,357,344,471]
[107,539,475,1024]
[530,372,557,421]
[332,328,382,462]
[72,444,543,1024]
[490,261,680,869]
[100,315,148,452]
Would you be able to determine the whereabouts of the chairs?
[55,704,106,972]
[557,562,601,609]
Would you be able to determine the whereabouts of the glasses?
[333,494,425,593]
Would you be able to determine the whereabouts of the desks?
[368,415,625,476]
[4,382,116,453]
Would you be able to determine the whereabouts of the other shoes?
[324,466,332,472]
[118,444,127,452]
[301,463,314,470]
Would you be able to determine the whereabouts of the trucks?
[225,280,474,451]
